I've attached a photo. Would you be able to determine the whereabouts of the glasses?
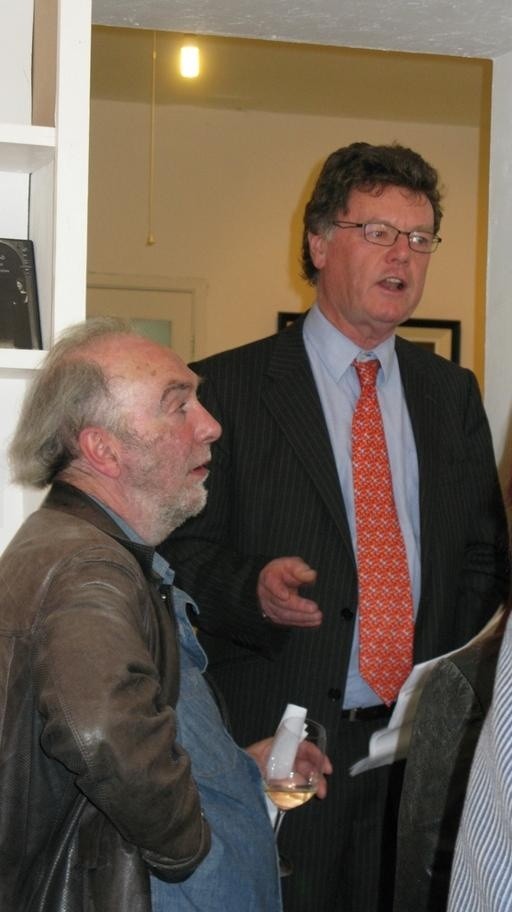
[330,216,444,257]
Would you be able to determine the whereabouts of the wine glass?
[262,716,329,879]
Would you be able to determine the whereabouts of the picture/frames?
[86,270,209,367]
[277,310,463,372]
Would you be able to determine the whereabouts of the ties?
[345,360,418,712]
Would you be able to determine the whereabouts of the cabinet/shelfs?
[0,123,58,371]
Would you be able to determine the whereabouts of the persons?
[2,319,334,912]
[148,150,512,912]
